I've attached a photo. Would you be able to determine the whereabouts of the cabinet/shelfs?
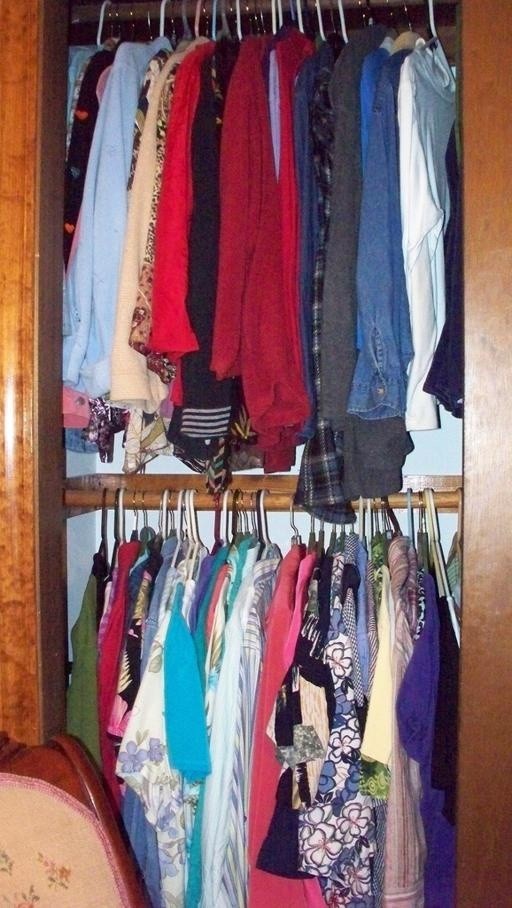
[0,0,512,908]
